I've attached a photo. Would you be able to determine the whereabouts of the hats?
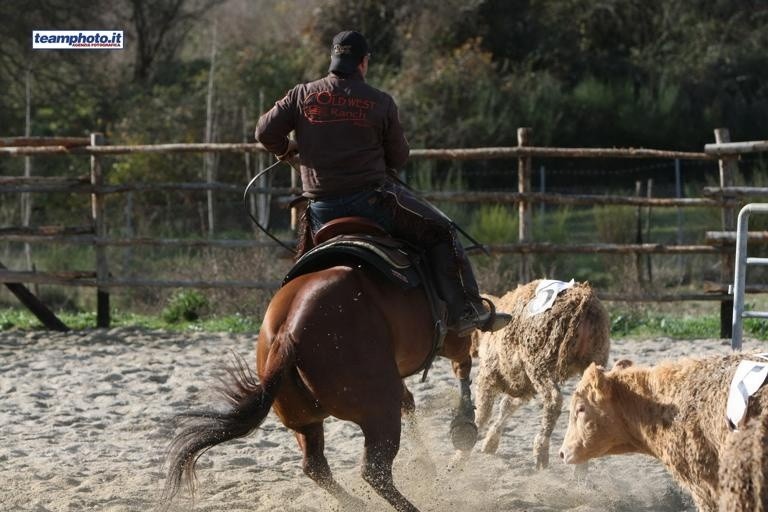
[327,31,367,73]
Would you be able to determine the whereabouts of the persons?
[254,29,513,337]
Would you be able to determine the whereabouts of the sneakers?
[456,306,513,338]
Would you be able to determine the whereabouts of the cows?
[558,353,768,512]
[470,279,611,474]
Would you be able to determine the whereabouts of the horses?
[159,262,479,512]
